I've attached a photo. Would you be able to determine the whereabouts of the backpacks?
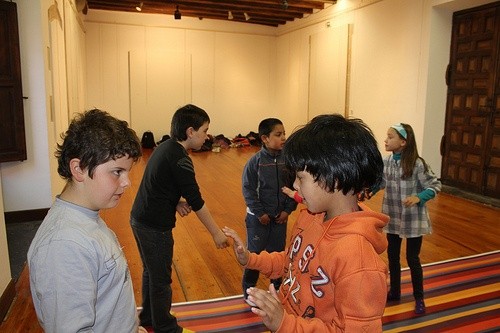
[141,130,156,149]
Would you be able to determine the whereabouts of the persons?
[359,123,442,313]
[242,118,298,308]
[281,186,305,205]
[223,114,390,333]
[27,109,146,333]
[130,104,231,333]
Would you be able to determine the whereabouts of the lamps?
[244,12,250,21]
[174,7,181,19]
[228,11,233,19]
[135,2,143,11]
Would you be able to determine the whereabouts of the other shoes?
[415,298,425,313]
[243,297,257,306]
[387,291,401,300]
